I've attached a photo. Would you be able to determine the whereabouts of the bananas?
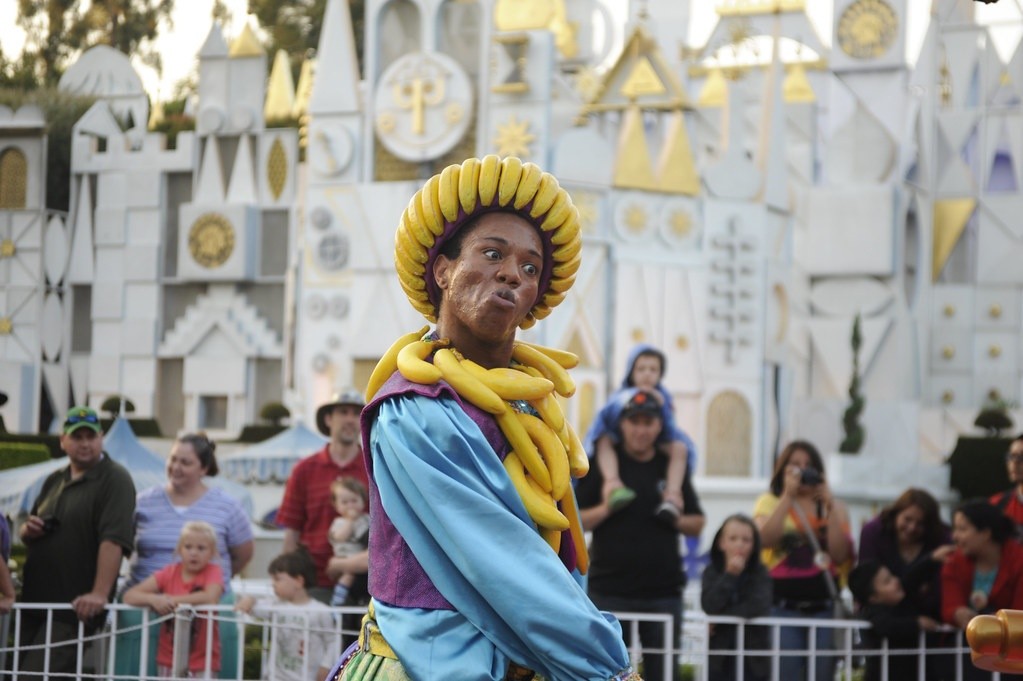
[363,326,590,575]
[392,155,582,329]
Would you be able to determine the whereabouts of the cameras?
[42,517,59,532]
[800,467,824,487]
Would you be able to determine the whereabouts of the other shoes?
[608,488,637,511]
[654,502,680,523]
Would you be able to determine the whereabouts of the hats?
[62,405,101,436]
[621,392,662,419]
[316,388,367,435]
[395,153,583,332]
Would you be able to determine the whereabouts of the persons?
[0,514,17,681]
[3,405,136,681]
[274,391,371,649]
[122,520,226,681]
[593,343,689,525]
[695,435,1023,681]
[316,155,640,681]
[105,430,257,681]
[569,384,705,681]
[325,477,373,605]
[240,550,342,681]
[328,549,370,577]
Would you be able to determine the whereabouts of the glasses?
[1004,452,1022,464]
